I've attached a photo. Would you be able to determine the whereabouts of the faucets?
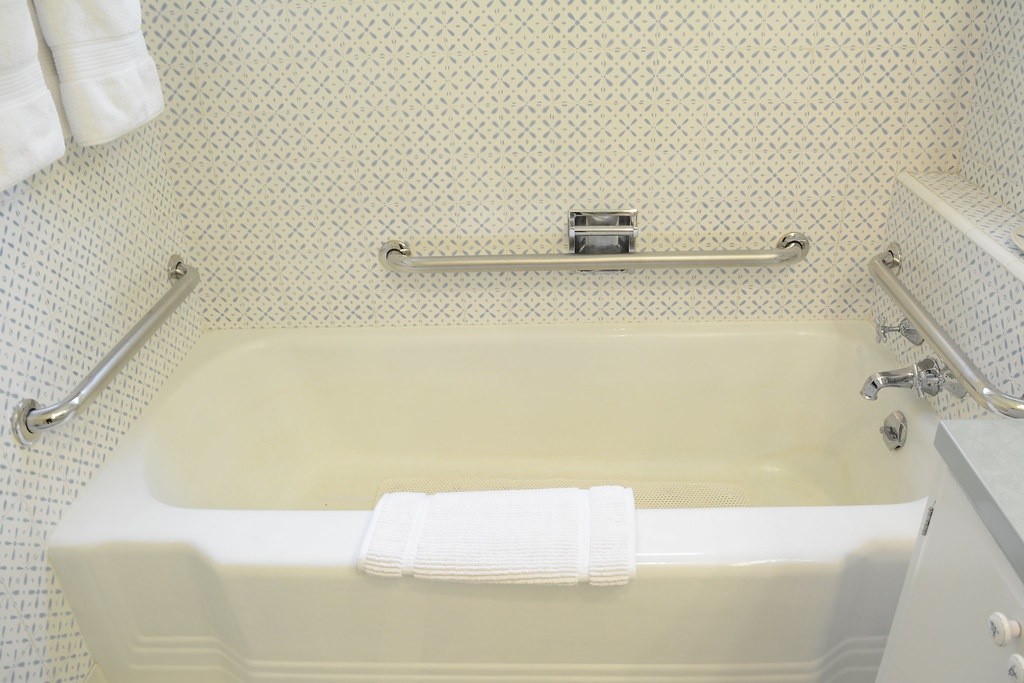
[858,356,944,402]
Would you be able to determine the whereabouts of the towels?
[33,0,168,152]
[1,0,69,200]
[354,484,640,592]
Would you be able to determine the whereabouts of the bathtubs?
[46,317,971,683]
